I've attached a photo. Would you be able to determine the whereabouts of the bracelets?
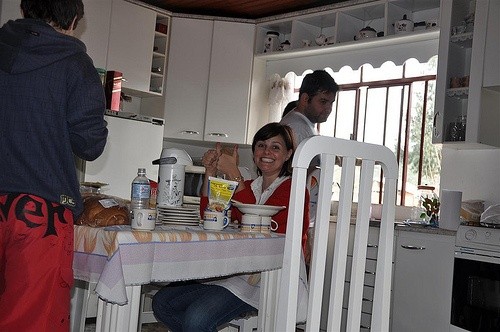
[228,176,244,182]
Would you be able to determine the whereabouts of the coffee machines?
[152,149,194,207]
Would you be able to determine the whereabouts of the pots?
[392,15,426,34]
[360,25,377,39]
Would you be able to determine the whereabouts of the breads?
[74,195,130,227]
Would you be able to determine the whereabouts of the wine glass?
[456,114,467,126]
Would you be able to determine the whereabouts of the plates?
[157,206,201,226]
[231,202,286,217]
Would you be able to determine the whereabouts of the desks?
[69,223,310,332]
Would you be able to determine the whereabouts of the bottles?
[411,184,435,222]
[96,68,106,87]
[130,168,151,224]
[263,31,289,53]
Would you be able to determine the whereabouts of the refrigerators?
[76,109,164,202]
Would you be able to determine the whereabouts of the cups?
[452,25,467,36]
[261,216,279,233]
[448,122,462,140]
[203,211,230,232]
[240,214,260,233]
[450,76,461,88]
[131,209,156,232]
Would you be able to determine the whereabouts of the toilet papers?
[440,190,462,230]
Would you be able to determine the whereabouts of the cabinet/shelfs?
[0,0,500,332]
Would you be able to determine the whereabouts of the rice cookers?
[460,202,485,223]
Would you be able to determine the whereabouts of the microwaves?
[183,165,206,204]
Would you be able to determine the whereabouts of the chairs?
[274,134,400,332]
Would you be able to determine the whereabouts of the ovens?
[449,252,500,332]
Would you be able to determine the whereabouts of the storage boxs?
[104,69,123,113]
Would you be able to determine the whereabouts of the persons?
[0,0,109,332]
[152,122,310,332]
[280,70,361,174]
